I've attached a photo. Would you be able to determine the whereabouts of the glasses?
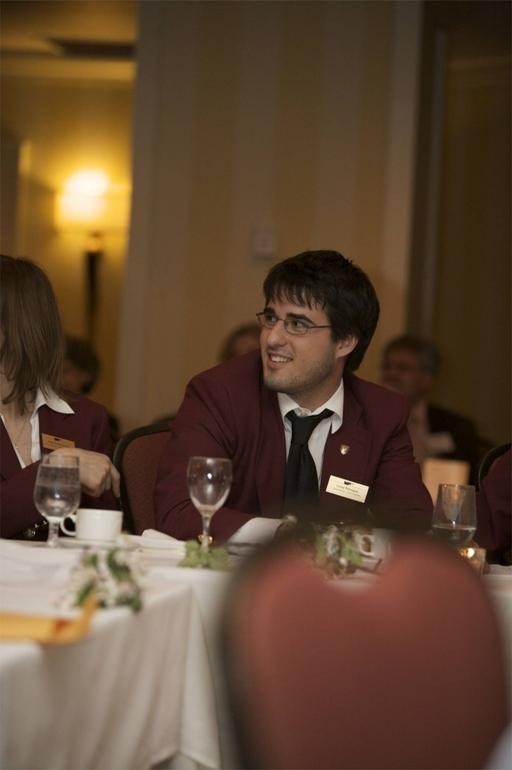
[256,311,331,337]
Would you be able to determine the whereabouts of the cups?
[60,508,124,547]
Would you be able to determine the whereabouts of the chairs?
[110,423,176,533]
[477,442,511,563]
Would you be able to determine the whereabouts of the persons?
[64,330,101,397]
[149,248,437,555]
[380,331,497,507]
[0,253,122,542]
[217,321,263,364]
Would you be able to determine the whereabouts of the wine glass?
[32,453,81,549]
[187,456,232,570]
[432,483,477,549]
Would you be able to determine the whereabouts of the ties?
[282,408,333,508]
